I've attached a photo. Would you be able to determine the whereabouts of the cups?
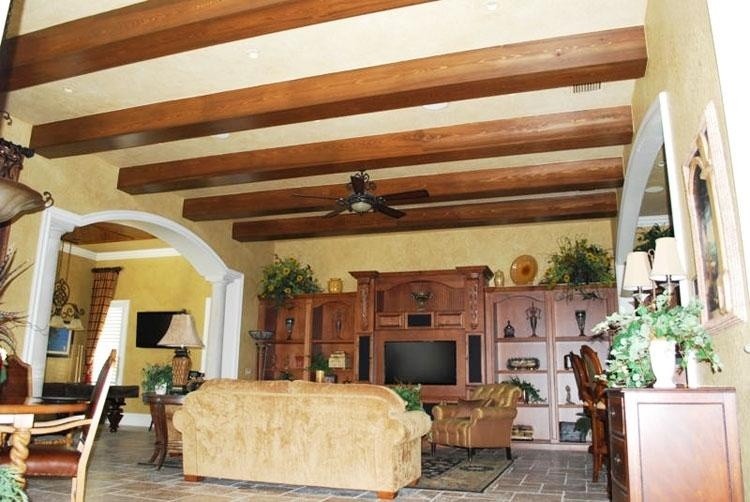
[564,355,573,370]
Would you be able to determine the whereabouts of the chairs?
[0,349,117,502]
[429,384,522,462]
[0,354,32,405]
[569,345,609,483]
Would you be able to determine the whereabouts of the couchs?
[172,377,432,500]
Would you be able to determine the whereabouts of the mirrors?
[615,92,700,387]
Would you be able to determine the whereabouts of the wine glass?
[574,309,588,336]
[286,319,294,341]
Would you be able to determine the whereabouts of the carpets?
[138,450,518,493]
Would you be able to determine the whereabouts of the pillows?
[451,397,492,419]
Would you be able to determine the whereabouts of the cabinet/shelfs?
[257,291,356,382]
[609,385,745,502]
[484,286,616,452]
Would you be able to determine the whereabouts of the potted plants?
[307,353,331,383]
[142,364,173,395]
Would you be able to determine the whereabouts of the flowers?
[634,224,671,251]
[537,236,614,302]
[259,253,321,308]
[591,290,724,388]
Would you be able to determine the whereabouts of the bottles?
[328,278,343,294]
[494,267,504,289]
[504,320,515,337]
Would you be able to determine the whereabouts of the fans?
[293,169,429,220]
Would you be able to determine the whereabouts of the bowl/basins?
[248,330,274,339]
[509,255,538,284]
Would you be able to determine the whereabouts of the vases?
[328,278,342,293]
[576,311,585,336]
[285,318,294,340]
[494,270,503,287]
[649,341,676,388]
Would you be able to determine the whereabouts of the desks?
[0,404,87,502]
[43,382,139,435]
[143,392,184,469]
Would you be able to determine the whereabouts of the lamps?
[48,242,85,330]
[157,308,205,395]
[623,236,684,305]
[248,330,274,380]
[0,109,53,223]
[351,202,371,215]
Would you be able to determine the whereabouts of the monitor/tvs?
[46,325,71,356]
[135,310,189,350]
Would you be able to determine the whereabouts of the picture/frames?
[47,330,74,358]
[559,421,586,443]
[681,101,746,335]
